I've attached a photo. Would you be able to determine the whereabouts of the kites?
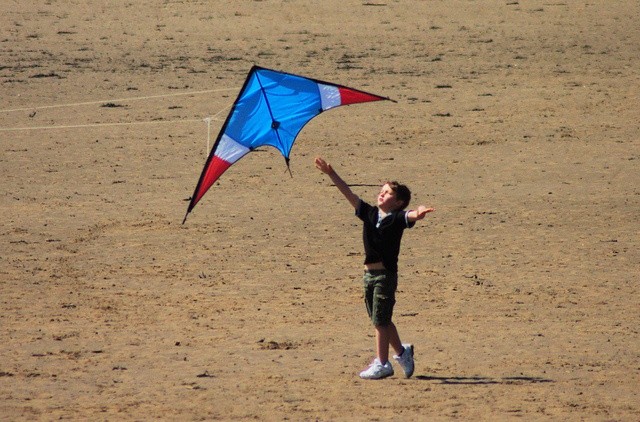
[181,63,399,225]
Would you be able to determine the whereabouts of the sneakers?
[359,358,394,379]
[393,344,414,377]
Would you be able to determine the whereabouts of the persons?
[314,156,435,379]
